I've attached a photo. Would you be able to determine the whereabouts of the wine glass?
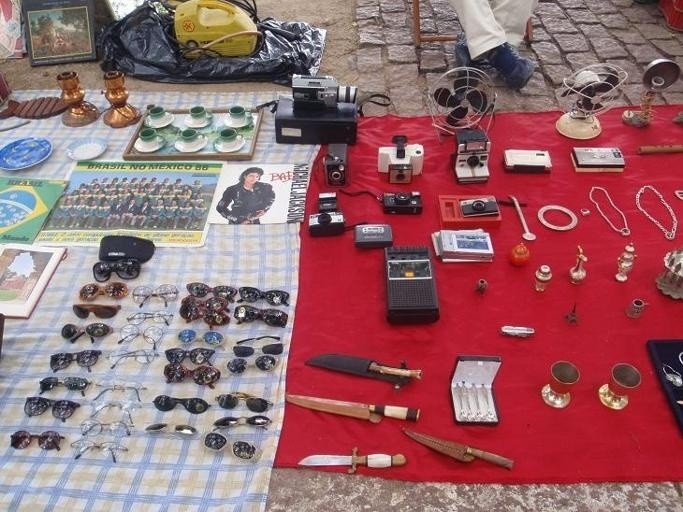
[538,360,579,408]
[596,364,641,409]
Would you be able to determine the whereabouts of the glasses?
[106,348,159,370]
[79,281,129,302]
[179,283,288,328]
[177,329,224,346]
[214,392,274,413]
[69,439,130,461]
[212,414,271,432]
[49,350,101,374]
[118,324,164,350]
[37,376,92,398]
[226,356,278,376]
[164,364,221,389]
[92,260,140,283]
[11,430,66,452]
[22,395,81,422]
[72,304,122,320]
[90,402,135,425]
[61,323,113,344]
[232,336,283,357]
[80,420,132,439]
[131,284,178,310]
[153,395,211,414]
[92,378,148,404]
[204,430,258,461]
[144,423,198,440]
[125,310,174,327]
[165,348,216,366]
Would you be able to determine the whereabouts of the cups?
[228,106,245,124]
[148,106,170,124]
[180,130,204,147]
[138,128,164,147]
[220,129,243,147]
[189,107,206,123]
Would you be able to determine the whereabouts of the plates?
[184,112,212,128]
[64,137,106,161]
[223,114,252,128]
[0,137,53,171]
[213,135,244,152]
[174,136,207,153]
[144,113,175,129]
[133,135,164,153]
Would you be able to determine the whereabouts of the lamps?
[623,59,680,126]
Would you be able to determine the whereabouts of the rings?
[581,208,590,216]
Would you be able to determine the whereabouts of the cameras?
[382,191,422,213]
[324,143,347,187]
[378,134,424,184]
[292,74,357,107]
[572,147,626,173]
[453,128,492,182]
[353,222,393,247]
[308,212,344,236]
[460,196,499,217]
[503,149,552,172]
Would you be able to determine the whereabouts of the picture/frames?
[24,1,99,67]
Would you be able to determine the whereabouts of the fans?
[555,62,628,141]
[427,66,498,143]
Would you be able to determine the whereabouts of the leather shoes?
[454,38,480,88]
[488,43,533,89]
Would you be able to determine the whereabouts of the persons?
[448,0,540,88]
[47,177,207,229]
[216,167,275,225]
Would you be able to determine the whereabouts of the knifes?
[301,354,424,389]
[295,445,406,473]
[283,394,420,424]
[400,427,513,470]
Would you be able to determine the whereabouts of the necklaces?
[590,187,630,236]
[636,186,678,240]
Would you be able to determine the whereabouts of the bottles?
[509,242,530,268]
[625,298,645,318]
[534,265,553,293]
